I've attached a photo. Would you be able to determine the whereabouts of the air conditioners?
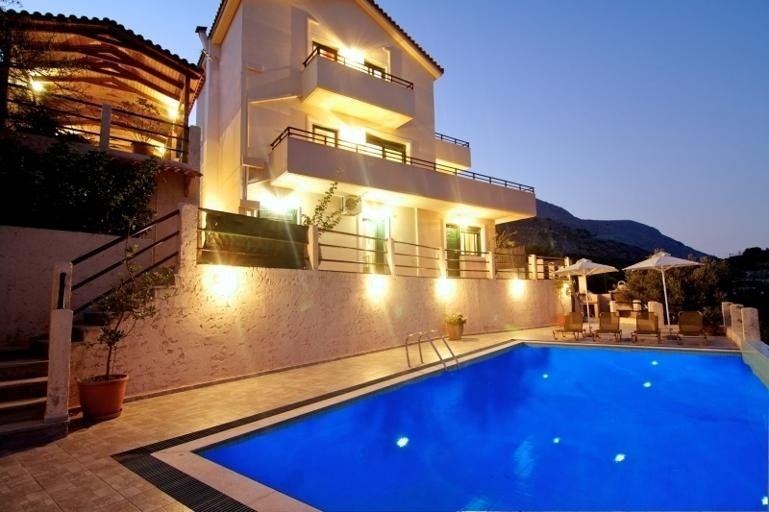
[339,195,362,216]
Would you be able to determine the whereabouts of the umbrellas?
[554,258,619,333]
[621,249,705,330]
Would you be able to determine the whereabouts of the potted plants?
[552,274,577,326]
[444,311,467,338]
[77,214,167,421]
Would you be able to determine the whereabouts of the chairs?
[675,310,707,345]
[631,313,660,345]
[592,310,621,341]
[553,313,585,343]
